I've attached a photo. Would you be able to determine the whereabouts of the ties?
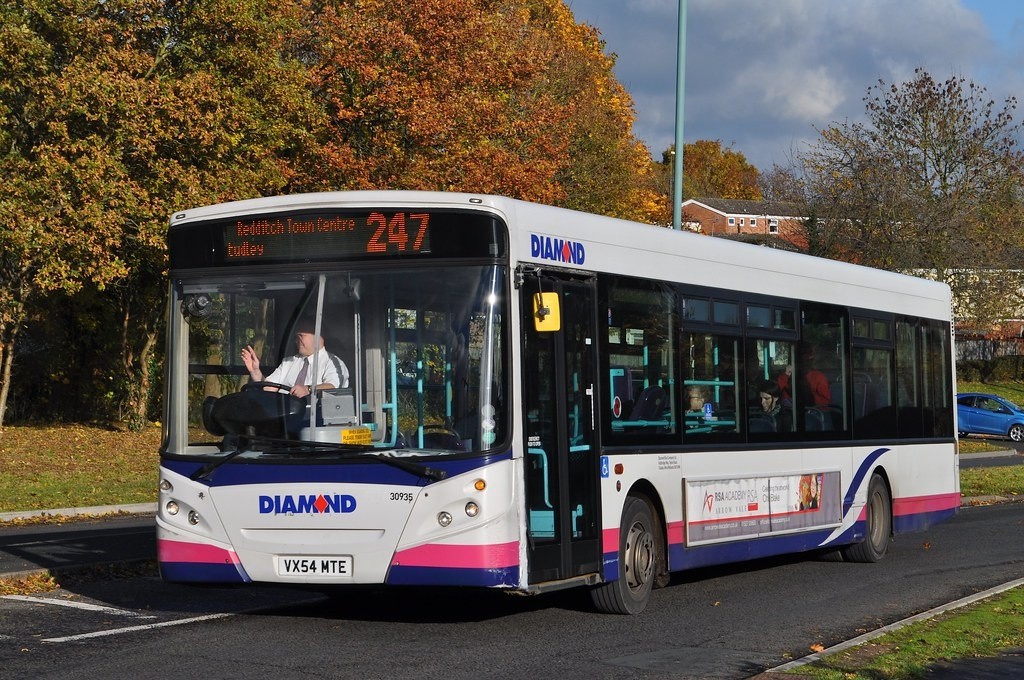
[295,358,309,386]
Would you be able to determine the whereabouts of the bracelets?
[308,386,312,394]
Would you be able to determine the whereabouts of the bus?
[153,189,965,614]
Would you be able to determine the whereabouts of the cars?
[957,393,1024,442]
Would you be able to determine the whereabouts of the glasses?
[686,396,699,400]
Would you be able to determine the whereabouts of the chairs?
[413,425,465,451]
[823,403,842,430]
[625,385,667,433]
[749,412,777,433]
[806,406,824,432]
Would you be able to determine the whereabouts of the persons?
[834,346,880,416]
[684,339,791,434]
[777,340,831,412]
[240,315,349,429]
